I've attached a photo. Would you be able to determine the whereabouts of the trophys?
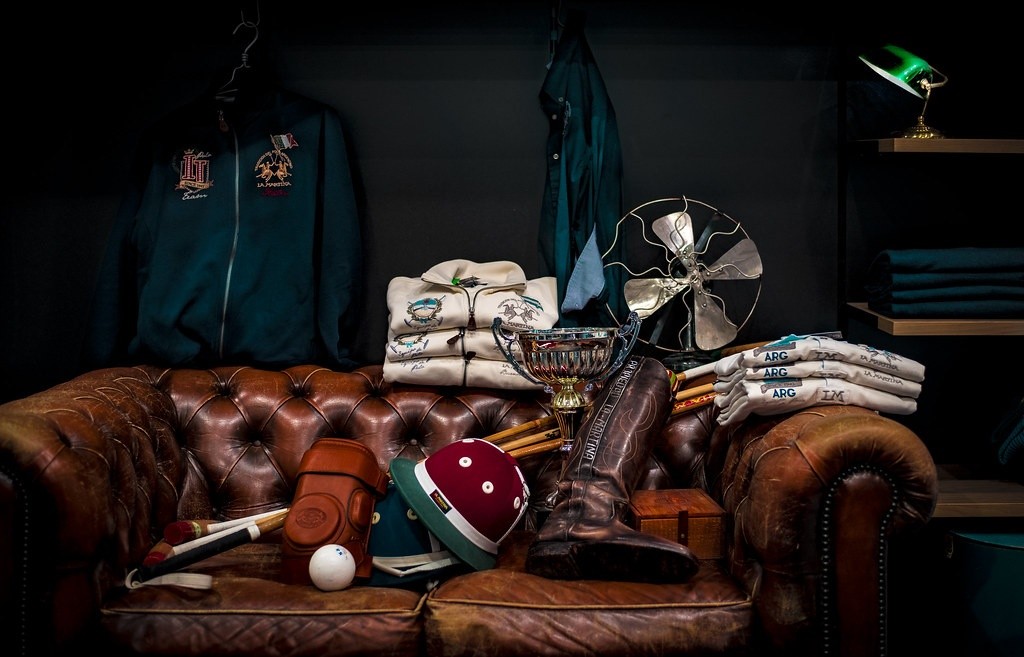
[491,311,642,534]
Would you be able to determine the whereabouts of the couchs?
[1,361,944,657]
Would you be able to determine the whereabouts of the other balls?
[309,543,356,592]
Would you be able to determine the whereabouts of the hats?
[389,437,532,571]
[370,491,460,585]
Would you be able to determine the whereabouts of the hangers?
[206,21,261,102]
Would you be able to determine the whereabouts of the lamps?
[859,41,950,141]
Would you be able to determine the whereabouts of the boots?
[527,353,701,581]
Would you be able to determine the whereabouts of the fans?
[598,193,765,349]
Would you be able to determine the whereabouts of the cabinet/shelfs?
[849,135,1024,522]
